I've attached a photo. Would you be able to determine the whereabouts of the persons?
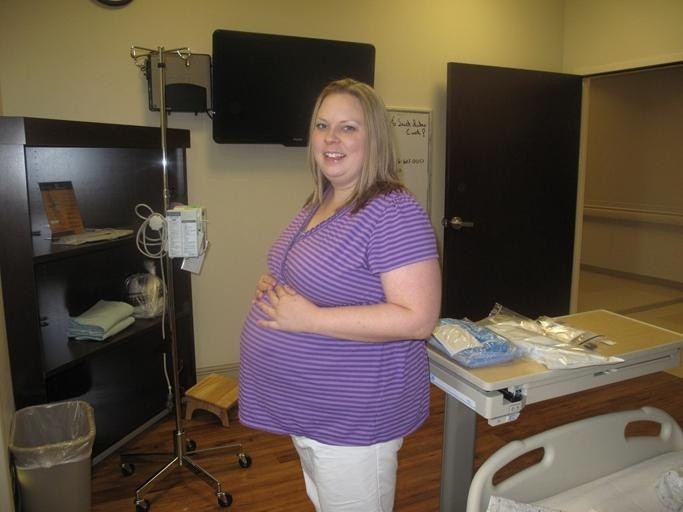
[238,77,443,512]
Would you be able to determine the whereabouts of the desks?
[418,309,683,512]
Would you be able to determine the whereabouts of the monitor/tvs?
[210,29,375,148]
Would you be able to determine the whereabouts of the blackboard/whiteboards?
[382,109,433,215]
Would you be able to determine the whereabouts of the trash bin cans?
[9,402,96,512]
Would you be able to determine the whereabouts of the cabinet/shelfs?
[1,116,197,472]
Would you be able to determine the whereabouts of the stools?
[184,375,239,427]
